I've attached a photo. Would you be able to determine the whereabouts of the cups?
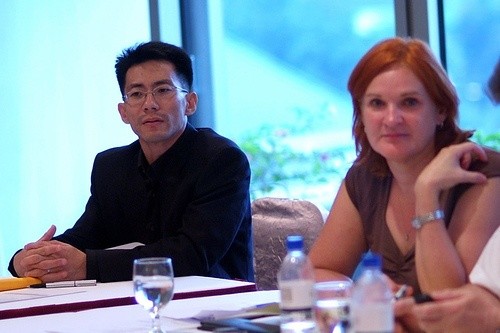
[311,278,351,332]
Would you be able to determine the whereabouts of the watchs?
[412,210,444,229]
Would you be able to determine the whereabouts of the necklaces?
[399,203,410,240]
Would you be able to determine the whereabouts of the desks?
[0,266,259,320]
[0,276,408,333]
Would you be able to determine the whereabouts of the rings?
[48,269,49,273]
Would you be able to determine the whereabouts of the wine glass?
[132,256,175,333]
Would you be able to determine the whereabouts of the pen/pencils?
[26,279,97,288]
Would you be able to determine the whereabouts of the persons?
[304,36,500,299]
[394,65,500,333]
[9,41,258,288]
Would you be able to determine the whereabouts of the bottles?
[350,254,395,333]
[278,234,317,333]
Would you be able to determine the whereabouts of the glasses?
[123,84,188,104]
